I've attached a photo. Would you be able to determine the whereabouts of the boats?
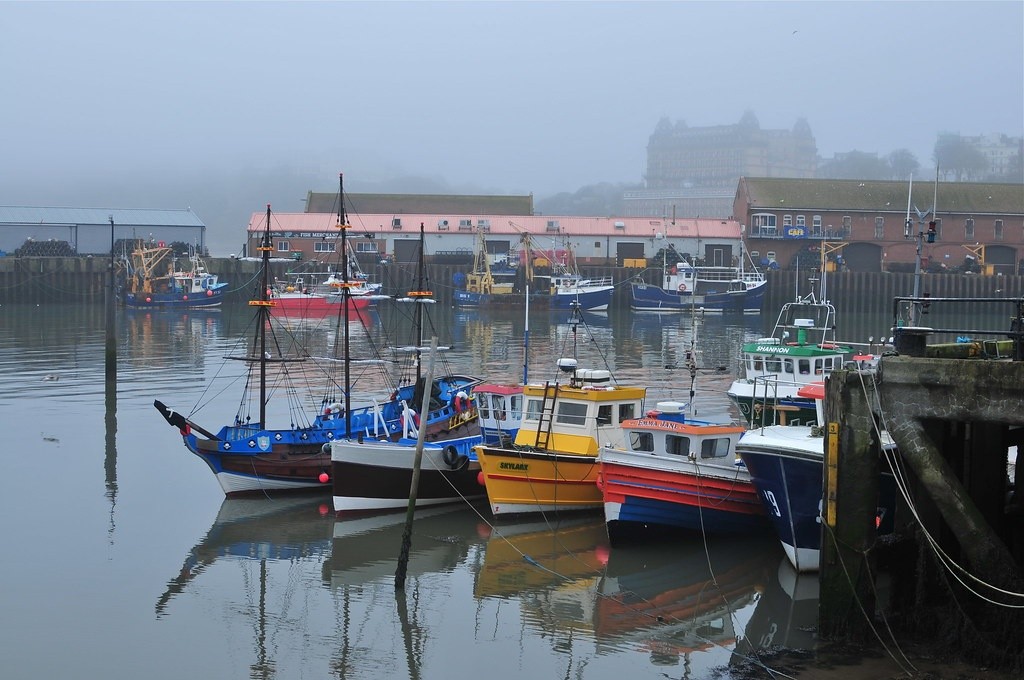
[627,222,768,314]
[589,535,786,680]
[725,548,874,680]
[325,227,904,580]
[153,484,333,680]
[111,232,230,312]
[321,500,489,680]
[451,224,616,313]
[468,507,610,600]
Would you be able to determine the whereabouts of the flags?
[153,397,188,434]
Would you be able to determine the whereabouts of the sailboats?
[147,171,496,499]
[258,237,379,311]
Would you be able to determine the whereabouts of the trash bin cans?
[890,327,934,359]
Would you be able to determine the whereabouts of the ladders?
[534,380,559,450]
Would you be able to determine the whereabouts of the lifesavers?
[817,344,839,349]
[646,410,658,419]
[470,286,477,292]
[529,296,535,302]
[399,408,420,438]
[324,402,345,420]
[679,283,686,290]
[443,444,470,475]
[565,281,571,288]
[787,342,808,346]
[391,386,406,400]
[582,385,615,390]
[455,390,471,420]
